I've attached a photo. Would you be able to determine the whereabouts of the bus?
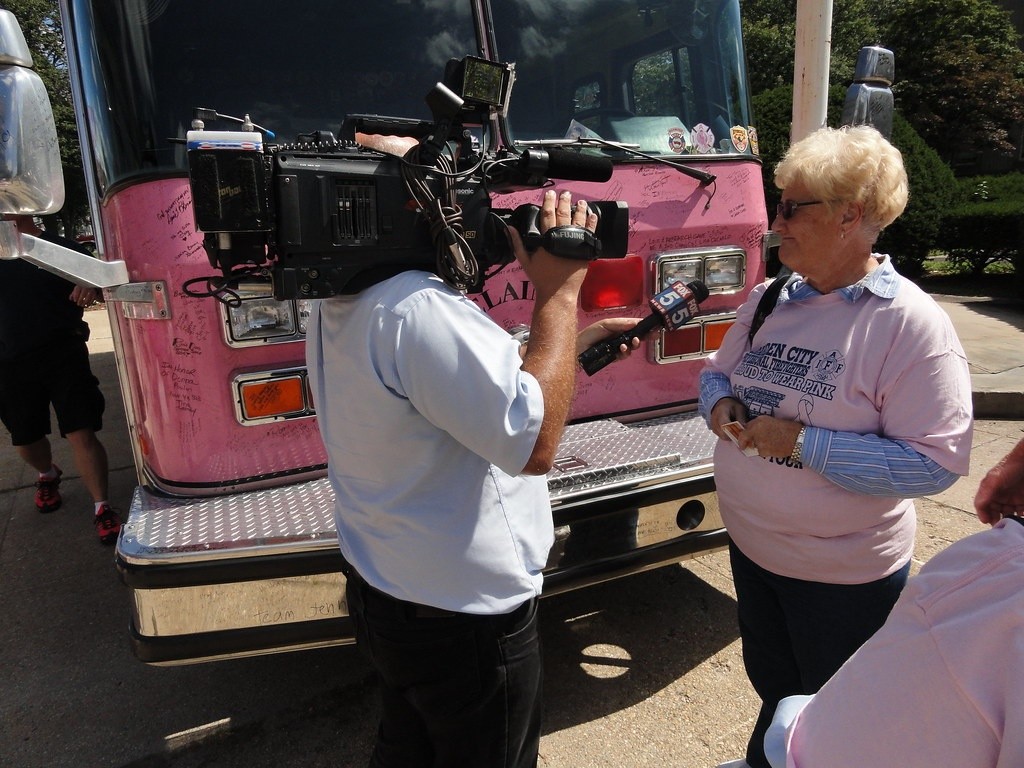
[1,0,896,680]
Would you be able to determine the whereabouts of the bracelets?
[788,424,807,463]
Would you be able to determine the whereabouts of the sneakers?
[95,503,122,545]
[34,463,63,514]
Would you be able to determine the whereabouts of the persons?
[0,211,127,547]
[284,134,666,766]
[666,122,971,767]
[757,429,1024,768]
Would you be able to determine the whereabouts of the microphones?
[578,281,709,377]
[519,149,613,182]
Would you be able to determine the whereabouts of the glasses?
[776,198,825,220]
